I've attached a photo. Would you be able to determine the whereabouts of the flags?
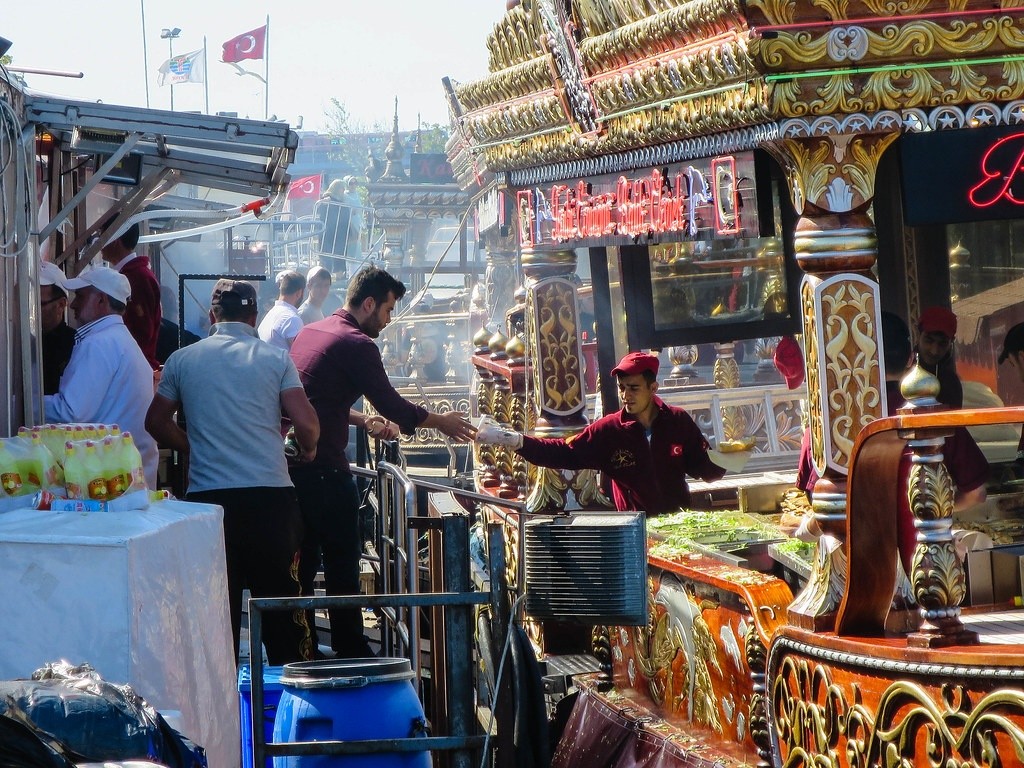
[222,26,267,64]
[284,173,324,199]
[156,49,204,87]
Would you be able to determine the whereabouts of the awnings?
[25,92,300,200]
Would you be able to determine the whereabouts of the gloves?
[499,423,523,451]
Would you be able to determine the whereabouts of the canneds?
[32,489,68,509]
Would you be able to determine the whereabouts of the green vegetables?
[646,509,816,562]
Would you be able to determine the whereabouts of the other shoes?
[335,643,376,659]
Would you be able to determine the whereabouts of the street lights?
[161,28,182,112]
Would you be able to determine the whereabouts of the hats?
[60,264,133,307]
[210,279,258,317]
[881,311,910,349]
[918,308,957,338]
[611,351,659,378]
[997,322,1024,366]
[774,335,805,390]
[35,259,70,303]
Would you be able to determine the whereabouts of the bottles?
[0,422,169,513]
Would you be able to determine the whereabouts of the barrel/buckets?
[272,658,435,768]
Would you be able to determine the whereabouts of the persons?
[475,353,729,517]
[11,173,485,664]
[775,306,1024,519]
